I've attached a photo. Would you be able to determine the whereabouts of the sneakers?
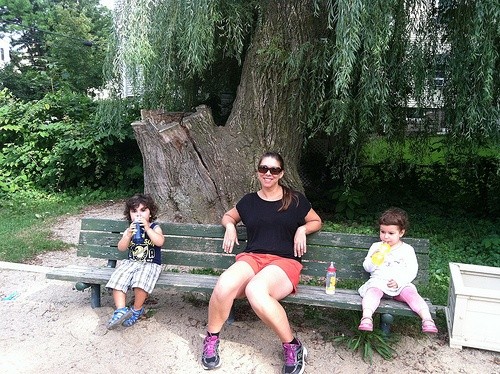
[201,332,221,370]
[282,337,307,374]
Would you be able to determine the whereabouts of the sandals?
[108,307,132,330]
[122,307,144,326]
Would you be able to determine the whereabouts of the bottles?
[372,242,391,266]
[325,261,336,295]
[133,217,146,245]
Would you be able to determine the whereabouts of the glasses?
[258,166,282,175]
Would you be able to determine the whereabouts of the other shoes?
[359,317,373,331]
[422,319,438,333]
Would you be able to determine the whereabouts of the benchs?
[47,218,437,336]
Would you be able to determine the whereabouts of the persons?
[105,194,165,329]
[201,151,321,374]
[358,208,438,334]
